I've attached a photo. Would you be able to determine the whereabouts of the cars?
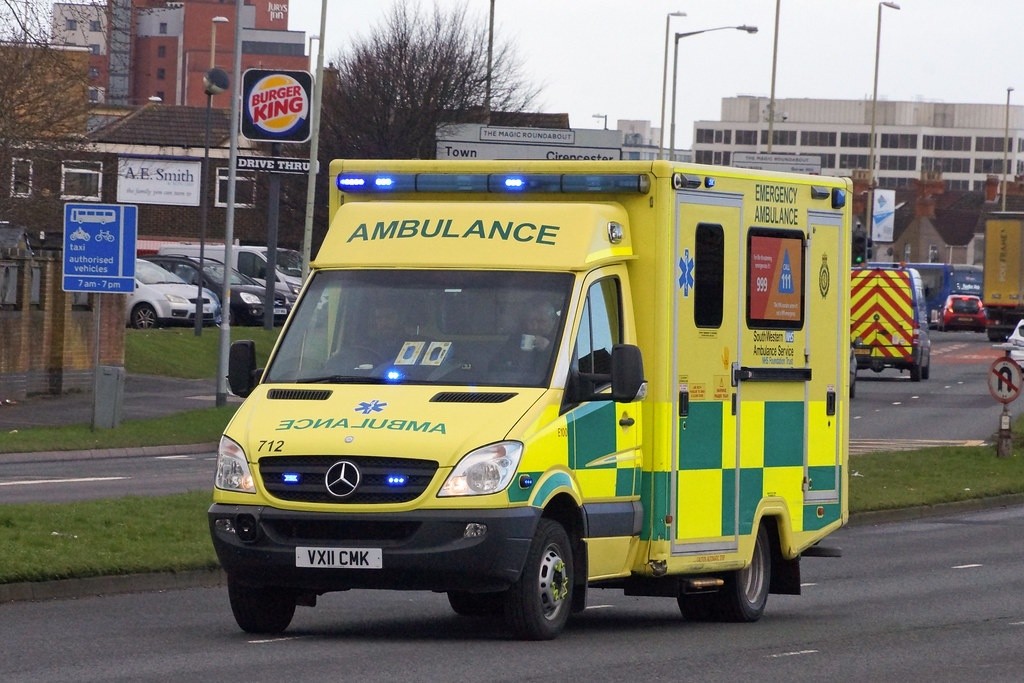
[138,253,293,327]
[126,258,223,327]
[943,293,987,328]
[1005,319,1024,371]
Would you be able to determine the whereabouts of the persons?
[490,298,582,403]
[325,296,437,380]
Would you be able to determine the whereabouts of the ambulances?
[209,156,851,639]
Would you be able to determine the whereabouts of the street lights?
[209,13,230,105]
[656,5,759,161]
[1001,86,1017,211]
[863,0,901,259]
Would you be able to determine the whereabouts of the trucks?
[158,243,312,307]
[982,210,1023,341]
[851,259,932,383]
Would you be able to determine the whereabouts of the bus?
[865,262,986,330]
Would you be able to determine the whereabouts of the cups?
[520,334,536,352]
